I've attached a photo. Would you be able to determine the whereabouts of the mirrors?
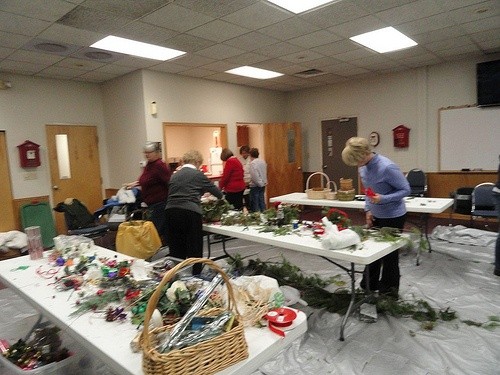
[162,123,229,178]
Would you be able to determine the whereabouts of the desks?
[203,210,413,340]
[1,237,307,374]
[268,192,455,266]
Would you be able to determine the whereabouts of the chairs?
[405,168,427,198]
[469,183,500,231]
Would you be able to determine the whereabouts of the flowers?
[48,245,153,321]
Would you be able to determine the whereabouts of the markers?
[473,168,481,171]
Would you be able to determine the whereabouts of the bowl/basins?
[264,307,296,326]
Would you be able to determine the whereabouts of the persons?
[121,141,171,238]
[340,136,412,300]
[247,147,268,212]
[163,148,225,277]
[218,148,246,210]
[238,145,253,186]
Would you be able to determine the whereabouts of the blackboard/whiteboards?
[438,105,500,171]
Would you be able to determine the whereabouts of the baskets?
[142,258,249,375]
[305,172,332,200]
[324,181,337,200]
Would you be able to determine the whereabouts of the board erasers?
[462,169,470,171]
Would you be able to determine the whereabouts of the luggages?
[455,187,474,214]
[471,182,499,217]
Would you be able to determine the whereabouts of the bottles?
[277,205,284,221]
